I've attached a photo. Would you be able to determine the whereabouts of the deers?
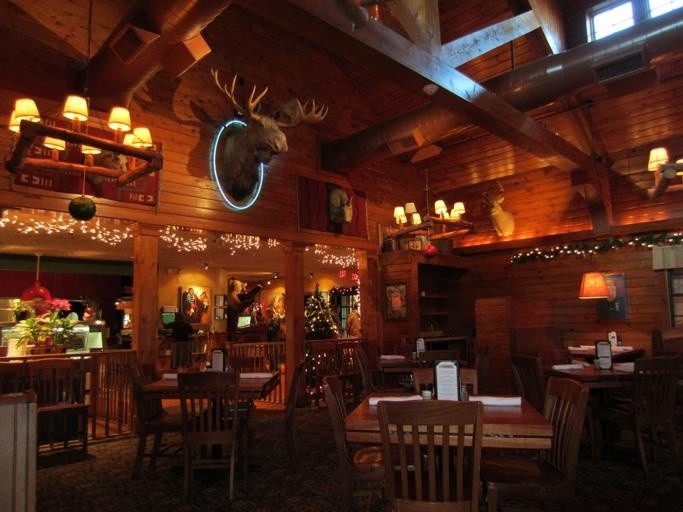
[210,67,330,202]
[481,180,515,237]
[329,189,353,224]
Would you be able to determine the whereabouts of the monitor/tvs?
[236,314,252,328]
[160,312,176,324]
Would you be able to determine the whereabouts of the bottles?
[412,352,417,362]
[225,365,231,373]
[593,359,599,370]
[460,386,468,401]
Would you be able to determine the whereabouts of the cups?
[421,390,431,400]
[420,291,425,296]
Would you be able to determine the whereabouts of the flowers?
[1,295,82,350]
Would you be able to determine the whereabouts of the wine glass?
[424,318,438,333]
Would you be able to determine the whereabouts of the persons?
[163,312,196,373]
[265,307,281,371]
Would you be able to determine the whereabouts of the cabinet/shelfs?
[379,251,473,365]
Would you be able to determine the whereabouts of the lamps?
[577,270,626,322]
[391,160,467,226]
[6,0,155,157]
[645,145,682,198]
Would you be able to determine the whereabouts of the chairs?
[321,337,681,512]
[28,359,88,459]
[129,358,308,502]
